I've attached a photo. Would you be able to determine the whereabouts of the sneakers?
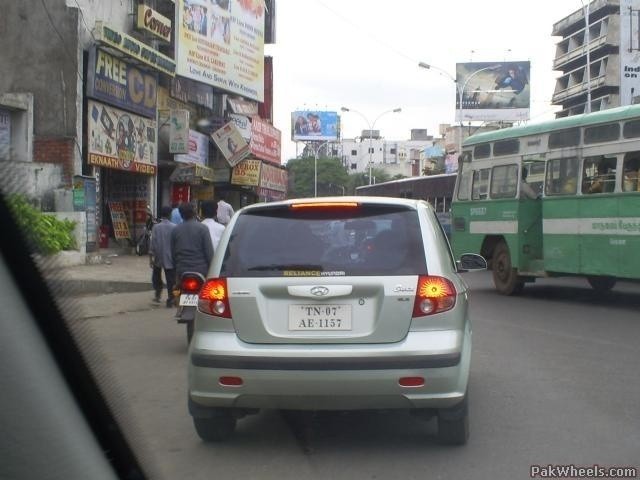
[151,297,161,306]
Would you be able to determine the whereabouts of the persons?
[90,108,155,162]
[226,137,239,154]
[516,166,540,199]
[142,189,235,310]
[548,158,640,195]
[306,113,322,136]
[295,115,308,136]
[494,65,530,95]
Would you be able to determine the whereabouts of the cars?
[181,196,489,446]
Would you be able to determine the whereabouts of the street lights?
[327,181,346,196]
[289,135,339,195]
[417,60,505,170]
[363,174,376,185]
[340,105,402,185]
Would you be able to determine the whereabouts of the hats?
[160,206,172,217]
[179,200,218,213]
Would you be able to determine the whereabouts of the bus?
[449,106,640,297]
[355,173,457,246]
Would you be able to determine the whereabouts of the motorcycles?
[179,270,207,344]
